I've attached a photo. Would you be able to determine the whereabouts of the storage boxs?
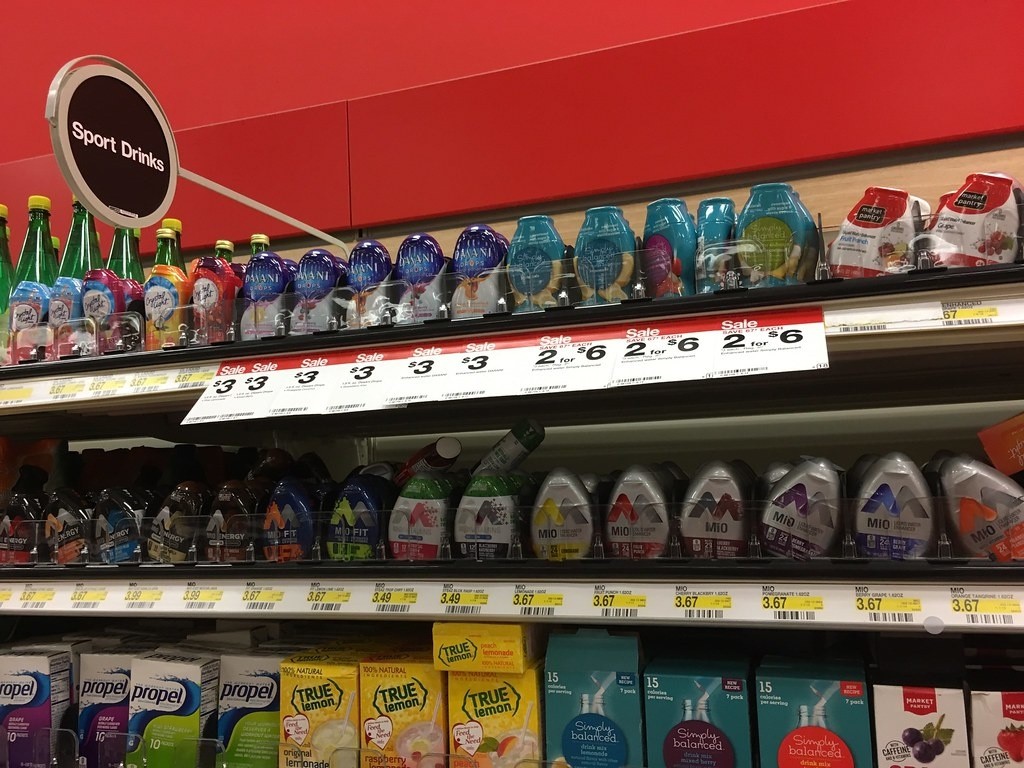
[0,622,1024,768]
[977,412,1024,476]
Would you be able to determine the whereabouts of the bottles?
[58,194,105,283]
[154,228,180,270]
[10,195,57,307]
[0,203,15,363]
[131,228,140,257]
[105,227,142,285]
[215,240,235,263]
[250,233,270,259]
[162,218,187,276]
[51,236,60,271]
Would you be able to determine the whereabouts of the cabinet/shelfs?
[0,262,1024,639]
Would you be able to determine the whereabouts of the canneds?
[8,259,234,361]
